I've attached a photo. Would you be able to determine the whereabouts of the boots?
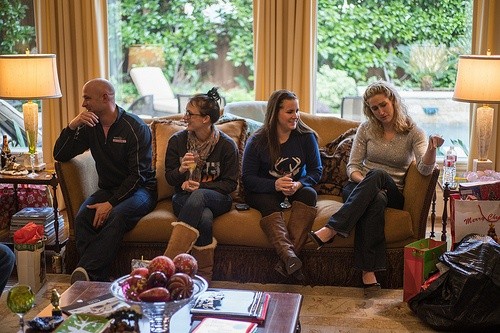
[273,201,318,276]
[162,221,199,260]
[259,212,302,275]
[190,238,217,280]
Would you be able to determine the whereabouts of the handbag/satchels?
[14,235,47,294]
[407,233,500,332]
[403,238,447,304]
[450,193,500,251]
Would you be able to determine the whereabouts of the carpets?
[0,233,452,333]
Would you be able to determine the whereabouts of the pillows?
[149,113,358,196]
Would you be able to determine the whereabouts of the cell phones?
[235,204,249,210]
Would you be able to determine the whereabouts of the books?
[10,206,65,244]
[52,292,130,333]
[188,291,270,333]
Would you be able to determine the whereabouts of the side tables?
[0,171,69,253]
[430,173,498,242]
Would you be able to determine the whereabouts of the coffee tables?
[19,281,303,333]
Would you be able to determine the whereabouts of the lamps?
[451,48,500,173]
[0,47,62,171]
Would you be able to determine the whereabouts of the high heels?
[360,272,382,290]
[308,231,335,249]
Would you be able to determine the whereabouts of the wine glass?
[7,285,37,333]
[186,150,198,189]
[280,171,293,208]
[25,130,39,177]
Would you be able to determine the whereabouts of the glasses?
[185,111,202,117]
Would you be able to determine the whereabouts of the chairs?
[130,66,181,113]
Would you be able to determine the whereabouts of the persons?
[53,79,158,286]
[308,82,445,296]
[241,90,322,280]
[0,242,15,297]
[161,87,240,286]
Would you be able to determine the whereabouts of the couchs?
[54,110,440,290]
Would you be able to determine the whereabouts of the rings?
[101,221,104,224]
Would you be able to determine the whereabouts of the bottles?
[1,135,11,169]
[443,146,457,188]
